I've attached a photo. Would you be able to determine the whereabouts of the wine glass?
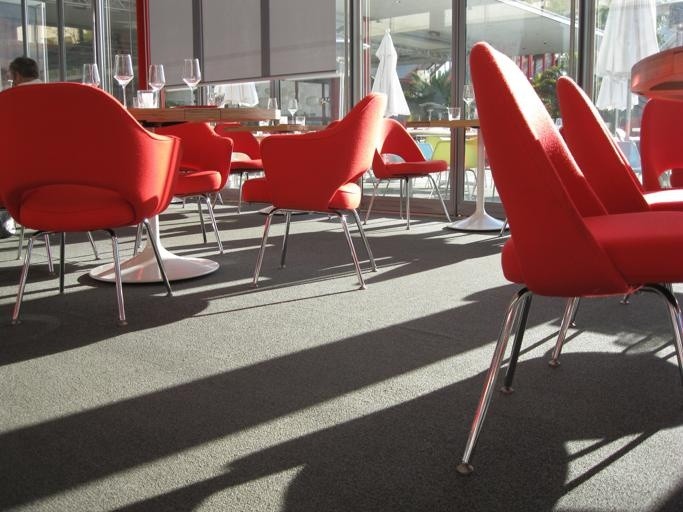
[267,96,278,126]
[181,58,201,105]
[148,64,165,108]
[286,98,299,124]
[113,53,134,106]
[462,84,475,119]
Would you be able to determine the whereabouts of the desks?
[403,118,514,233]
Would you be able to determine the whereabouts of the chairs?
[211,123,265,215]
[239,88,384,296]
[369,117,455,234]
[456,38,683,475]
[126,122,235,259]
[0,81,176,328]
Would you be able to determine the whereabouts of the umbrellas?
[214,81,259,107]
[595,78,639,137]
[594,0,661,140]
[372,29,412,119]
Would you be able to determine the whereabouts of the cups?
[556,118,562,127]
[136,89,156,109]
[295,115,305,126]
[447,107,461,121]
[82,62,101,89]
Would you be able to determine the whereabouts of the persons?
[9,56,42,87]
